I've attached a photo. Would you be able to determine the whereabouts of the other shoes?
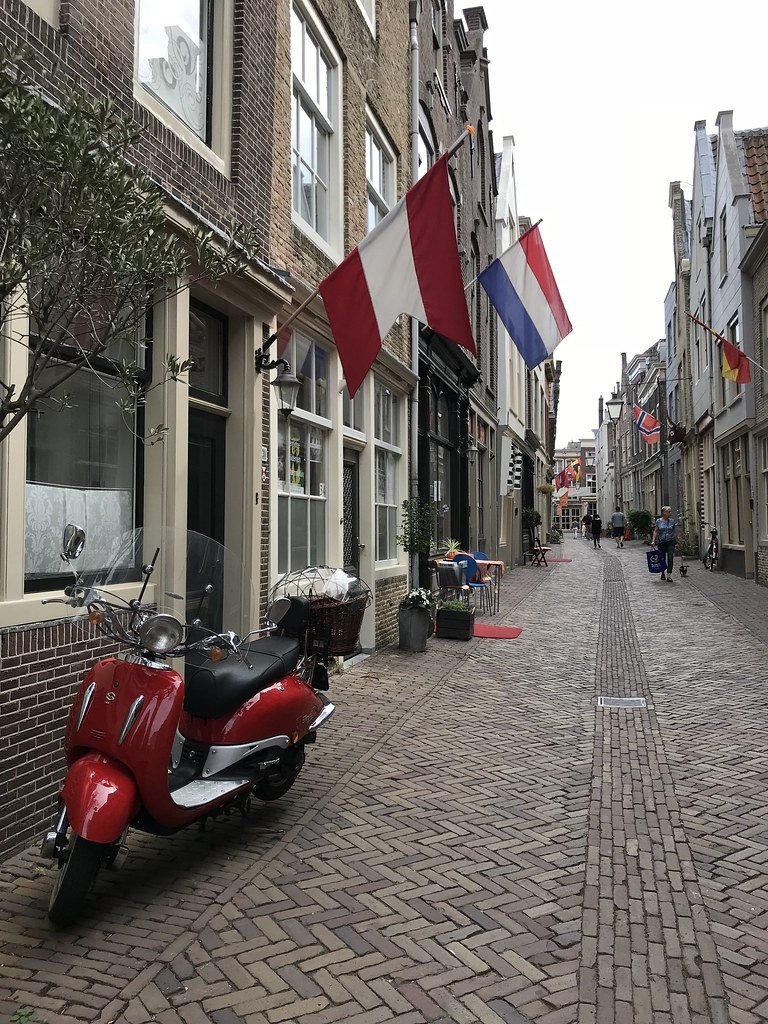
[598,543,601,548]
[593,546,596,549]
[621,542,624,547]
[616,545,620,548]
[666,577,673,582]
[661,574,666,580]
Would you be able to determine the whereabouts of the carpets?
[536,558,572,562]
[433,619,522,639]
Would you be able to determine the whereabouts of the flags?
[559,492,568,506]
[555,468,569,493]
[478,225,573,372]
[690,315,751,383]
[567,456,583,483]
[633,404,660,444]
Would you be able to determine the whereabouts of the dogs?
[679,565,690,576]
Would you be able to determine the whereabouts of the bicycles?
[704,525,719,572]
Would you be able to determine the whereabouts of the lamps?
[256,353,302,423]
[466,439,478,466]
[605,376,692,428]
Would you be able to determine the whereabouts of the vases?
[399,608,429,653]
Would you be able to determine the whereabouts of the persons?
[570,518,580,539]
[651,506,679,582]
[611,506,628,548]
[582,511,592,537]
[590,514,602,549]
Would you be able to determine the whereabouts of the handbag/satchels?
[646,546,667,573]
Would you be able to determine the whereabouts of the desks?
[443,559,505,616]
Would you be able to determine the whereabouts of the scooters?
[38,525,338,922]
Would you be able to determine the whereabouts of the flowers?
[398,587,440,611]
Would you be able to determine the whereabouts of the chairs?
[530,537,551,567]
[434,552,493,617]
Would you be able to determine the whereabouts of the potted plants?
[438,537,462,559]
[538,483,555,494]
[436,600,476,642]
